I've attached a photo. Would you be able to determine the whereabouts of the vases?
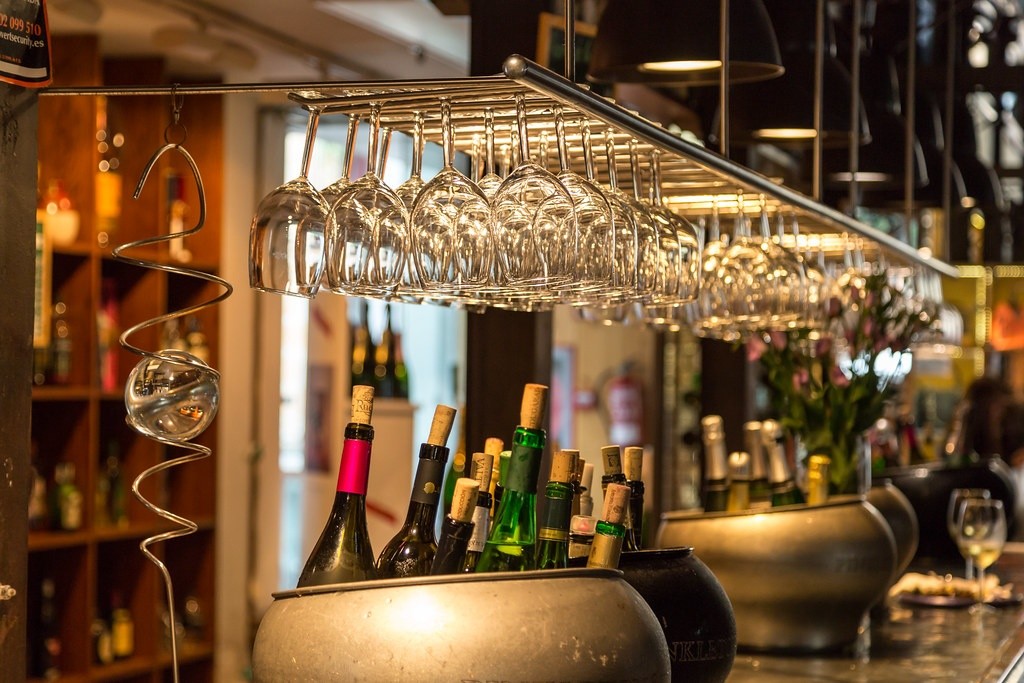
[808,433,874,495]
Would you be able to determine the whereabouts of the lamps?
[586,0,1004,213]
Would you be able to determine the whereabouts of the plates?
[902,592,979,607]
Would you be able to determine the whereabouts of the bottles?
[702,413,833,515]
[25,441,127,531]
[32,573,136,678]
[296,384,645,589]
[349,298,405,397]
[34,123,209,390]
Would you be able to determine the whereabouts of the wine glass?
[948,488,1009,615]
[245,85,887,344]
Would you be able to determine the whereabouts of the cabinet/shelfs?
[24,35,227,682]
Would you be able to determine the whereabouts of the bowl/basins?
[656,453,921,650]
[620,544,738,683]
[252,567,672,683]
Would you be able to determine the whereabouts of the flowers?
[739,267,945,493]
